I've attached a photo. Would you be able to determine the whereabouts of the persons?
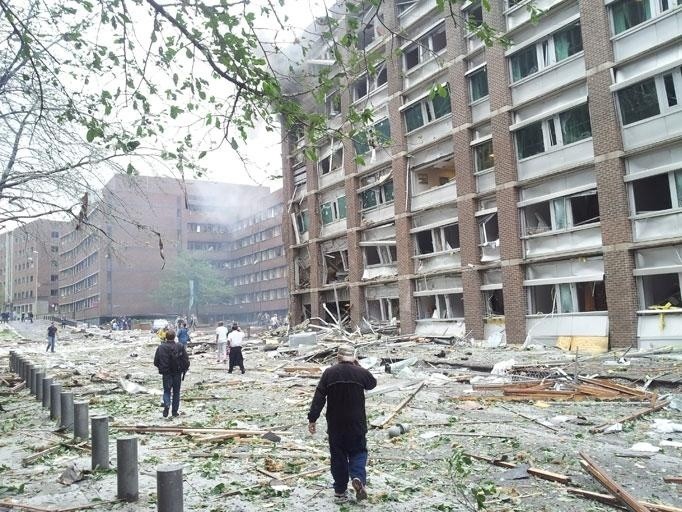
[257,312,281,328]
[62,317,67,329]
[307,343,377,504]
[110,312,132,330]
[0,311,33,324]
[45,321,58,352]
[174,313,198,331]
[154,329,191,417]
[176,322,189,349]
[215,321,246,374]
[156,324,171,345]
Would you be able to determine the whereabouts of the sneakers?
[163,405,169,417]
[352,476,368,500]
[333,491,349,504]
[172,411,179,416]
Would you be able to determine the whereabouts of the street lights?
[28,250,39,320]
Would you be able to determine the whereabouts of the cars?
[151,318,170,334]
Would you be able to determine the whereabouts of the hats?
[166,328,176,340]
[336,343,361,366]
